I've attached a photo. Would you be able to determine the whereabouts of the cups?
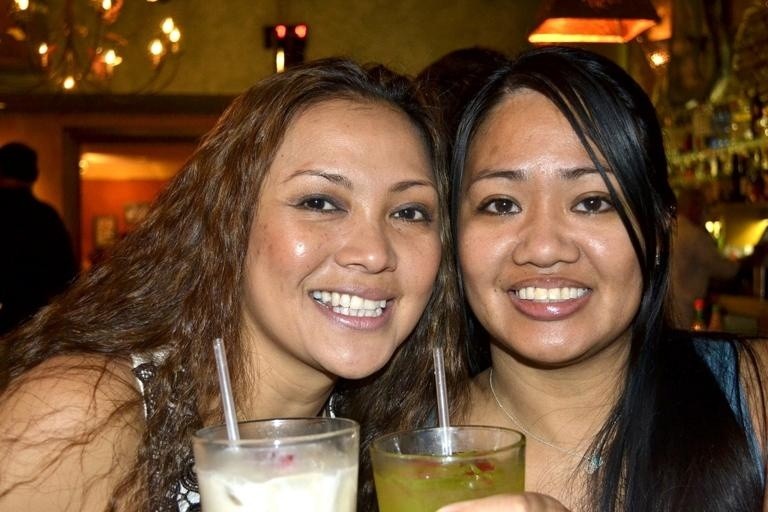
[191,416,360,512]
[369,425,526,512]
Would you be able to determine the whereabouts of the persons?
[1,143,77,338]
[1,56,462,512]
